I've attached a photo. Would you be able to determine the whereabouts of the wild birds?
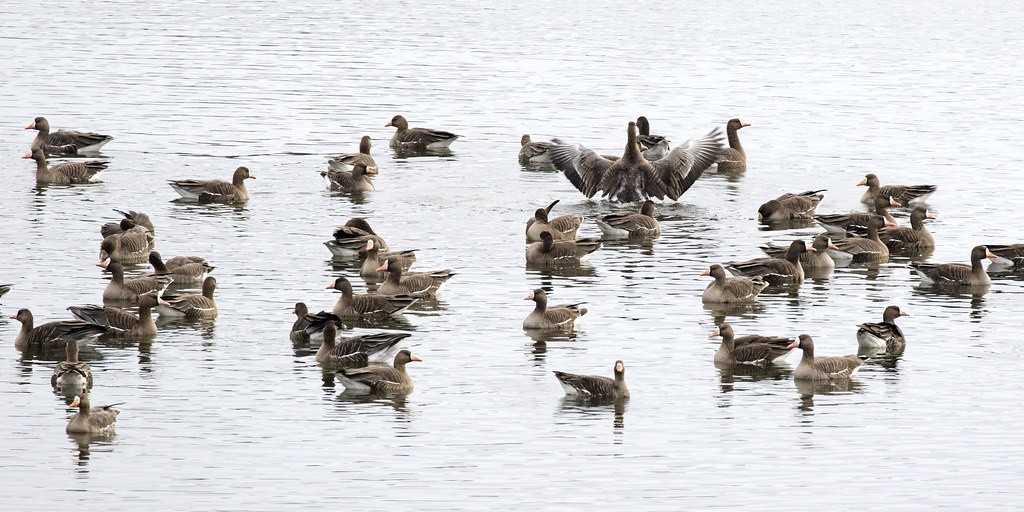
[908,245,997,286]
[290,218,457,401]
[560,395,630,431]
[523,288,589,329]
[24,117,115,156]
[320,136,379,193]
[552,360,630,398]
[721,194,937,287]
[758,189,828,221]
[786,334,871,380]
[0,208,220,434]
[20,148,111,184]
[385,114,465,153]
[856,173,938,208]
[700,264,770,303]
[165,166,257,204]
[983,244,1024,265]
[709,323,795,365]
[518,116,751,264]
[856,306,910,348]
